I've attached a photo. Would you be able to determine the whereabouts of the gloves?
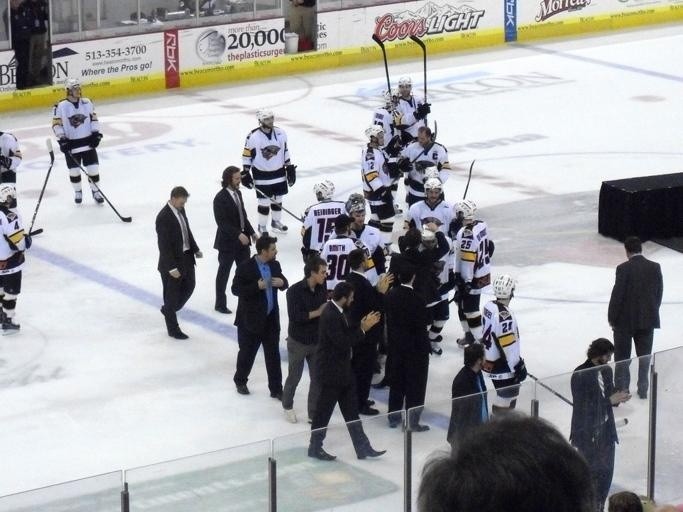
[414,102,432,119]
[516,358,527,381]
[377,188,392,202]
[286,164,298,187]
[22,233,31,249]
[398,157,413,173]
[387,162,398,178]
[0,155,12,171]
[58,137,71,154]
[88,133,103,148]
[240,171,254,190]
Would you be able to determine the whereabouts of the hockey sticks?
[68,152,132,222]
[410,35,428,128]
[371,33,393,116]
[462,160,476,200]
[249,183,305,223]
[526,372,629,428]
[391,120,437,184]
[27,138,55,235]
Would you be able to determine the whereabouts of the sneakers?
[258,224,267,235]
[92,190,104,203]
[456,338,467,346]
[430,341,442,355]
[282,404,297,424]
[75,191,82,203]
[307,418,313,424]
[0,312,21,330]
[271,220,288,231]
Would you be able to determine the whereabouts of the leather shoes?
[358,447,386,459]
[215,306,232,314]
[407,424,429,432]
[271,390,285,399]
[390,414,402,428]
[309,449,337,461]
[356,405,378,415]
[161,308,167,316]
[168,332,190,340]
[367,399,375,406]
[237,383,251,395]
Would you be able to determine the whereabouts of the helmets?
[0,185,16,203]
[346,194,365,213]
[420,230,435,241]
[385,88,399,103]
[424,166,439,178]
[256,106,274,121]
[424,177,443,192]
[454,200,478,220]
[314,180,334,200]
[365,125,384,140]
[398,76,412,87]
[334,214,355,226]
[494,275,515,299]
[66,77,81,91]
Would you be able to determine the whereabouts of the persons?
[398,126,450,206]
[288,1,316,52]
[385,255,429,432]
[330,193,388,288]
[392,77,430,146]
[370,223,449,389]
[407,177,458,242]
[453,201,490,350]
[608,236,665,400]
[282,259,330,424]
[0,183,32,334]
[348,253,395,416]
[213,166,258,314]
[362,124,400,261]
[482,273,526,415]
[320,214,385,299]
[21,1,49,85]
[231,239,288,402]
[0,133,22,182]
[371,92,403,214]
[302,180,345,260]
[446,343,488,450]
[53,76,104,208]
[179,0,213,15]
[570,338,631,511]
[156,187,202,338]
[306,282,387,461]
[418,229,457,354]
[418,412,599,511]
[606,492,642,512]
[4,0,36,89]
[241,108,297,235]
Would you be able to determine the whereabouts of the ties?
[477,376,487,425]
[598,369,605,399]
[178,212,190,248]
[343,312,352,360]
[234,193,244,229]
